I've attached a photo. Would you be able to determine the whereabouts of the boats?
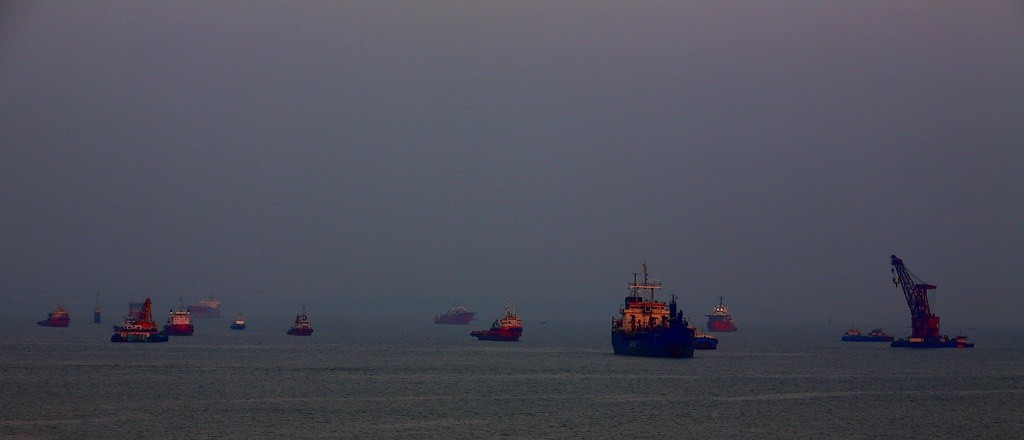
[94,292,102,324]
[188,291,222,318]
[840,328,894,342]
[36,303,71,327]
[162,296,195,336]
[230,313,247,330]
[694,332,720,351]
[706,297,738,332]
[111,296,170,343]
[287,304,314,336]
[434,304,477,326]
[469,297,525,342]
[890,335,974,348]
[611,261,696,359]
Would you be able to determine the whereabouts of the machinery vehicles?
[889,255,941,338]
